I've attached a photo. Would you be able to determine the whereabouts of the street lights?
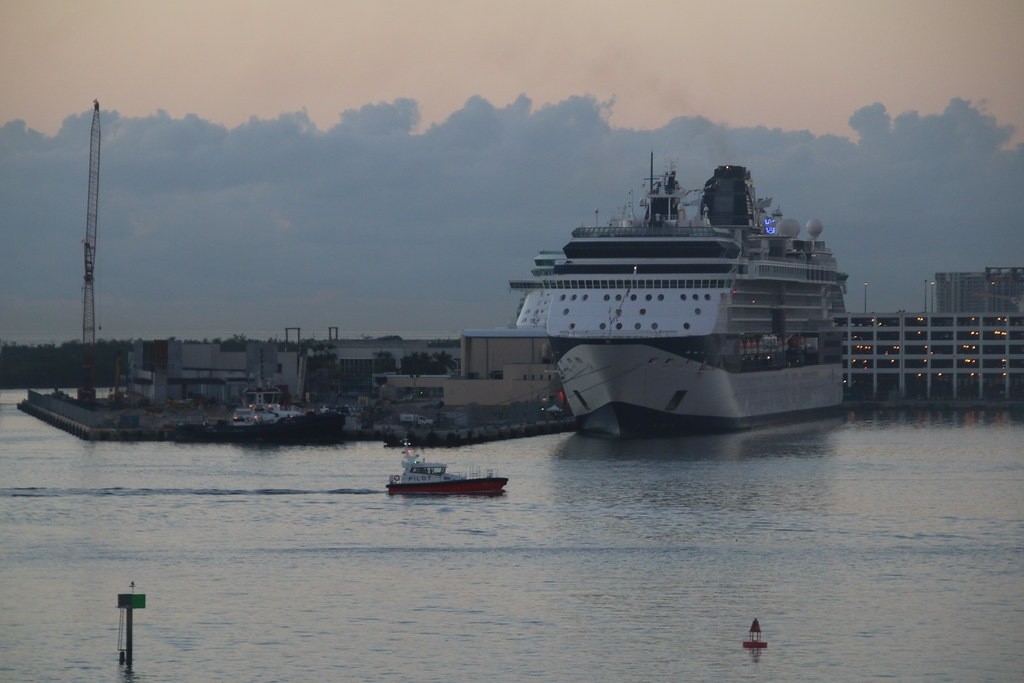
[931,281,934,312]
[864,281,867,313]
[924,280,927,314]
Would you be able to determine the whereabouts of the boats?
[386,457,509,498]
[510,163,846,440]
[173,379,346,439]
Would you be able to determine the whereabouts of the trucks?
[400,414,433,428]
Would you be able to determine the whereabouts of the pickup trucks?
[320,404,336,412]
